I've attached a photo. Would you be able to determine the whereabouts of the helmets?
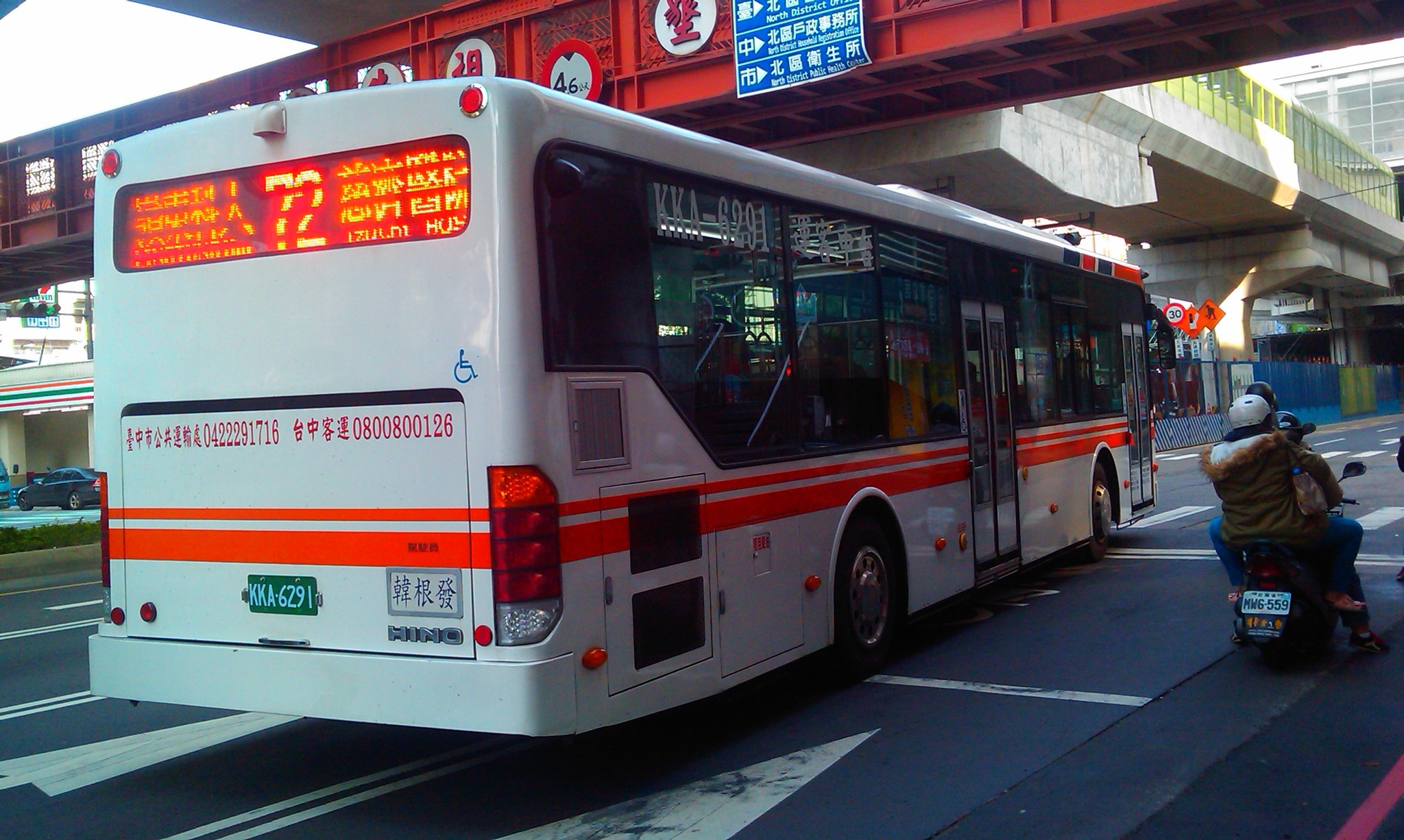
[1275,410,1303,444]
[1228,395,1271,430]
[1245,381,1276,406]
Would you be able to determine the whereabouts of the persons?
[1198,381,1391,656]
[1396,436,1404,578]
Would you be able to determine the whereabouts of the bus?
[84,73,1160,746]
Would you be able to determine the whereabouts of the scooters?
[1232,461,1367,667]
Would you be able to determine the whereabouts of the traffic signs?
[731,0,873,99]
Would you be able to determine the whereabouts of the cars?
[15,466,102,511]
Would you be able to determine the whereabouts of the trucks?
[0,458,19,510]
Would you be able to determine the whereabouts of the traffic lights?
[10,301,61,319]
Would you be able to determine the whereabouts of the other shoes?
[1228,592,1243,601]
[1328,593,1365,611]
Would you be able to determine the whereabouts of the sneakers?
[1355,631,1391,653]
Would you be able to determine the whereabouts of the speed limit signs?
[1163,303,1187,327]
